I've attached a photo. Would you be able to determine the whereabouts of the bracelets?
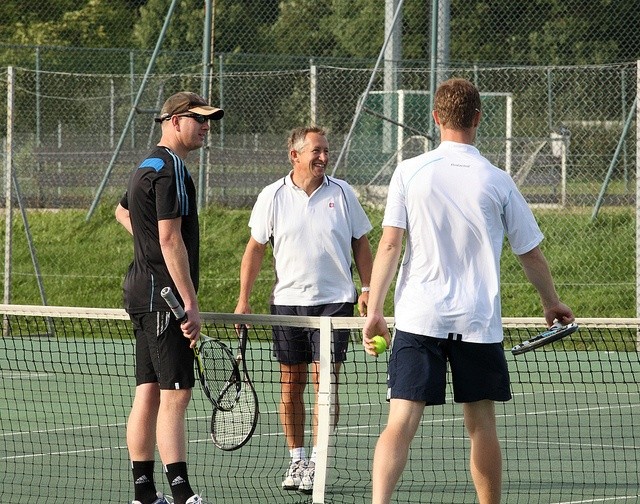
[361,286,370,292]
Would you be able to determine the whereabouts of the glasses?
[179,114,214,123]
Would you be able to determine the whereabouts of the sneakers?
[282,462,307,490]
[299,463,316,494]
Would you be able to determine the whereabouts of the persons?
[114,90,225,504]
[232,125,375,494]
[363,77,576,504]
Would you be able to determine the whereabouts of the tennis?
[368,335,388,354]
[356,302,367,314]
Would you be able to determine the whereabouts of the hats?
[160,93,224,120]
